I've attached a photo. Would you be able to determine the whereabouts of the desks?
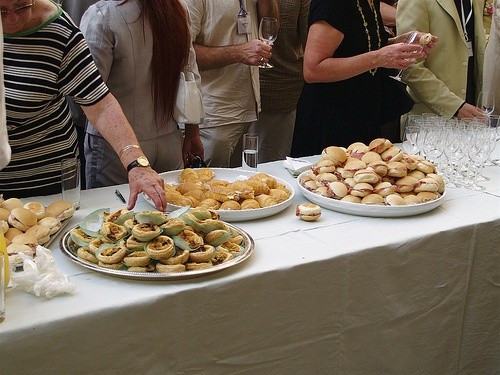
[0,127,500,375]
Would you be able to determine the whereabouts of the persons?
[290,0,439,158]
[396,0,500,141]
[80,0,192,190]
[185,0,274,168]
[249,0,311,164]
[0,0,167,211]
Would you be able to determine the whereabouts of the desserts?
[70,206,243,274]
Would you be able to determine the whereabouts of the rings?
[260,58,264,62]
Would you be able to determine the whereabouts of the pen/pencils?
[115,189,126,203]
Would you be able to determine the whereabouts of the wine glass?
[255,16,278,69]
[388,31,426,84]
[402,113,500,191]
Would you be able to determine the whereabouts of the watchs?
[127,156,149,172]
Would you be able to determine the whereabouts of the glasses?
[1,0,36,17]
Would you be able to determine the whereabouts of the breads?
[0,194,75,262]
[163,168,290,209]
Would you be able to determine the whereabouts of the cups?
[241,133,259,171]
[476,90,495,115]
[0,251,6,322]
[61,158,81,210]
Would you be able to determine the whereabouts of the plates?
[140,168,295,221]
[59,221,255,281]
[297,169,446,216]
[13,216,73,267]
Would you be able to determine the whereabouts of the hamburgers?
[296,203,321,221]
[301,138,445,206]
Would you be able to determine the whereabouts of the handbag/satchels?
[184,155,208,169]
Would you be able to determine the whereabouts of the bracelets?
[119,144,139,158]
